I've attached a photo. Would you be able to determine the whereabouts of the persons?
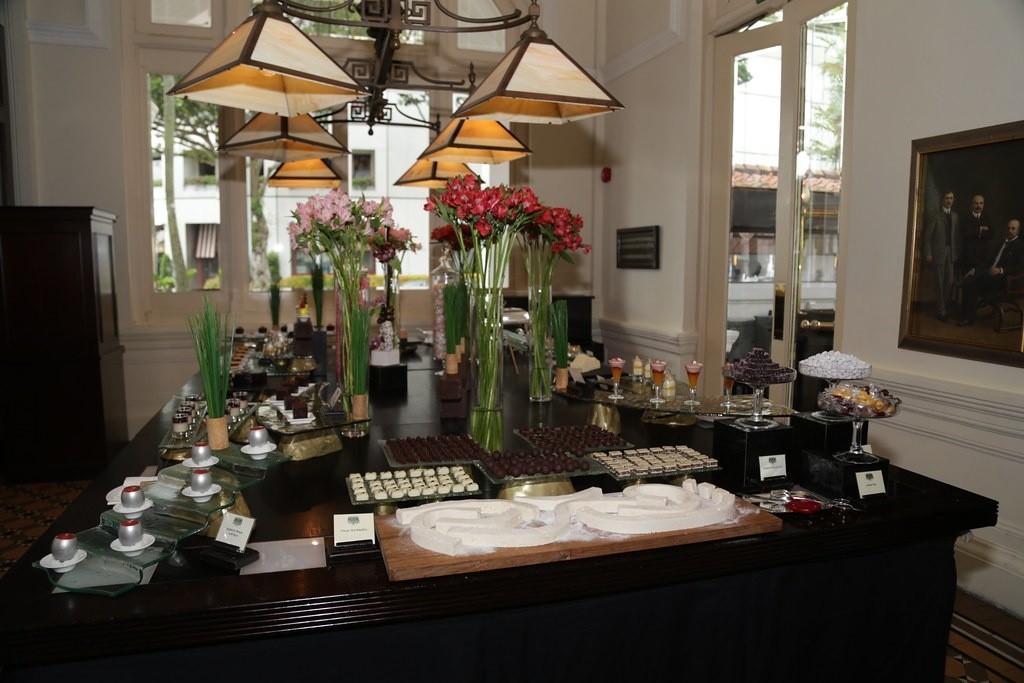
[921,189,958,323]
[964,217,1024,326]
[957,193,994,327]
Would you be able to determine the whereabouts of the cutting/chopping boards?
[375,494,783,582]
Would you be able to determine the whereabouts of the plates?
[227,402,261,437]
[109,534,155,558]
[113,499,154,519]
[272,400,316,425]
[242,442,277,460]
[182,456,222,503]
[40,549,89,573]
[158,404,208,449]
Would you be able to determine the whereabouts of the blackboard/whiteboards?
[616,225,660,270]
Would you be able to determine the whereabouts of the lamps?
[268,97,484,191]
[217,60,532,164]
[164,1,624,127]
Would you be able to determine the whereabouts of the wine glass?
[721,364,737,407]
[650,363,666,404]
[683,364,703,406]
[608,360,625,399]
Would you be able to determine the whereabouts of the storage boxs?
[794,408,870,452]
[709,419,796,487]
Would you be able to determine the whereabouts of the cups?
[224,391,247,431]
[171,393,204,440]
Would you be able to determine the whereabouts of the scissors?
[736,489,853,511]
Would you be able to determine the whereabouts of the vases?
[336,272,553,449]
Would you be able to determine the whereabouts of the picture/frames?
[898,121,1024,371]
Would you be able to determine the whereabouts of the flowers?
[293,180,596,285]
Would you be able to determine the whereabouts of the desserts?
[731,348,790,384]
[348,425,736,556]
[275,387,308,419]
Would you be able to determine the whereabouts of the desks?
[1,322,1000,683]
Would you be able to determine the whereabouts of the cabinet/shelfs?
[0,204,128,475]
